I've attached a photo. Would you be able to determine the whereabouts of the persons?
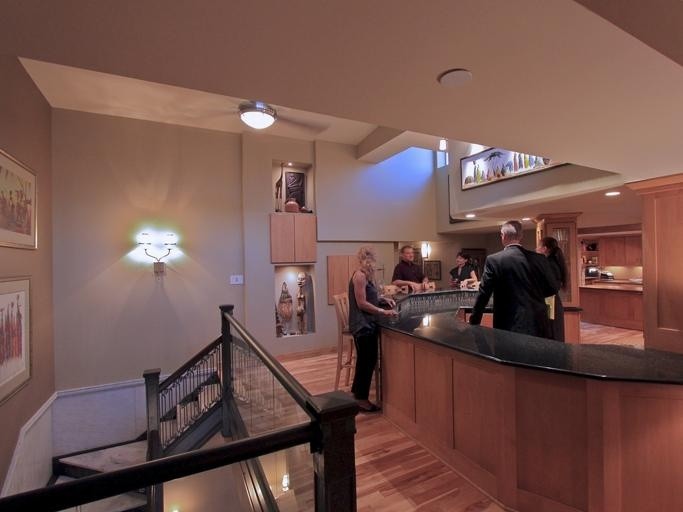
[448,251,477,289]
[348,246,398,412]
[392,245,431,292]
[469,220,569,342]
[295,272,307,333]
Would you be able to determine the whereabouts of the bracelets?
[378,307,384,317]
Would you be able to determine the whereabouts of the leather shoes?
[353,401,381,413]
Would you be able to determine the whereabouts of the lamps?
[135,231,178,277]
[238,107,279,130]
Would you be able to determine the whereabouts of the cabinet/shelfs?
[269,213,318,264]
[600,235,643,266]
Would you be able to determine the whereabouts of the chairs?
[332,292,380,404]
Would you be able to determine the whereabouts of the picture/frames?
[0,147,40,251]
[0,276,33,404]
[460,148,570,191]
